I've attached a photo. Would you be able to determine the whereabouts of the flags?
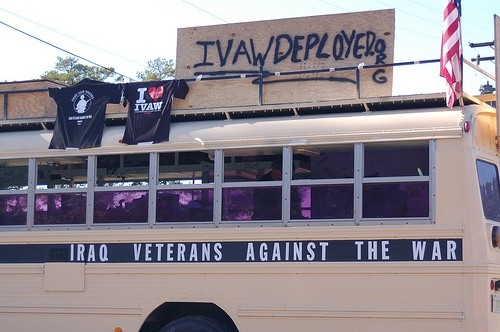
[440,1,465,109]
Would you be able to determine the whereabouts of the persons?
[251,155,305,218]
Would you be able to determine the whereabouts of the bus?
[0,56,500,332]
